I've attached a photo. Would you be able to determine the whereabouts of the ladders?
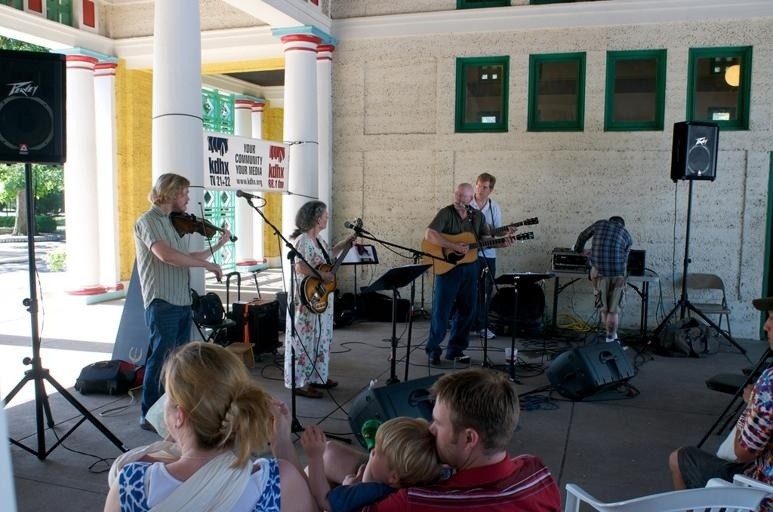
[171,210,237,242]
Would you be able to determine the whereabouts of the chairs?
[446,353,470,363]
[361,420,381,453]
[295,387,323,398]
[430,352,440,365]
[310,380,337,388]
[480,328,496,339]
[607,336,629,351]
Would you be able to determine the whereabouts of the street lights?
[76,360,144,395]
[193,293,225,324]
[658,318,712,358]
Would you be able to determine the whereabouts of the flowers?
[236,190,258,198]
[344,222,369,236]
[460,201,475,215]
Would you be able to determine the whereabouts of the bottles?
[752,298,772,311]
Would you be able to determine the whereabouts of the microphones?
[225,343,256,369]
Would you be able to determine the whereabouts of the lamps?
[696,347,773,448]
[686,274,731,347]
[565,477,768,512]
[733,474,773,512]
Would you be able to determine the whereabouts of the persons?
[423,182,517,366]
[667,295,773,511]
[299,415,441,512]
[304,366,562,512]
[103,340,320,511]
[283,200,357,400]
[446,171,519,340]
[573,215,633,345]
[133,173,230,434]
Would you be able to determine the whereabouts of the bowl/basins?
[179,455,213,461]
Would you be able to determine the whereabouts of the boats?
[229,300,280,354]
[0,50,66,164]
[671,122,719,183]
[546,340,638,401]
[349,372,446,455]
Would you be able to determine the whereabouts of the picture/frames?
[547,264,659,336]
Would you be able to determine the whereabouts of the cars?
[504,347,518,364]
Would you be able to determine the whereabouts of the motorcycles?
[267,258,352,447]
[652,180,746,356]
[0,164,129,463]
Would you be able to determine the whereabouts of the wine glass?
[279,401,290,415]
